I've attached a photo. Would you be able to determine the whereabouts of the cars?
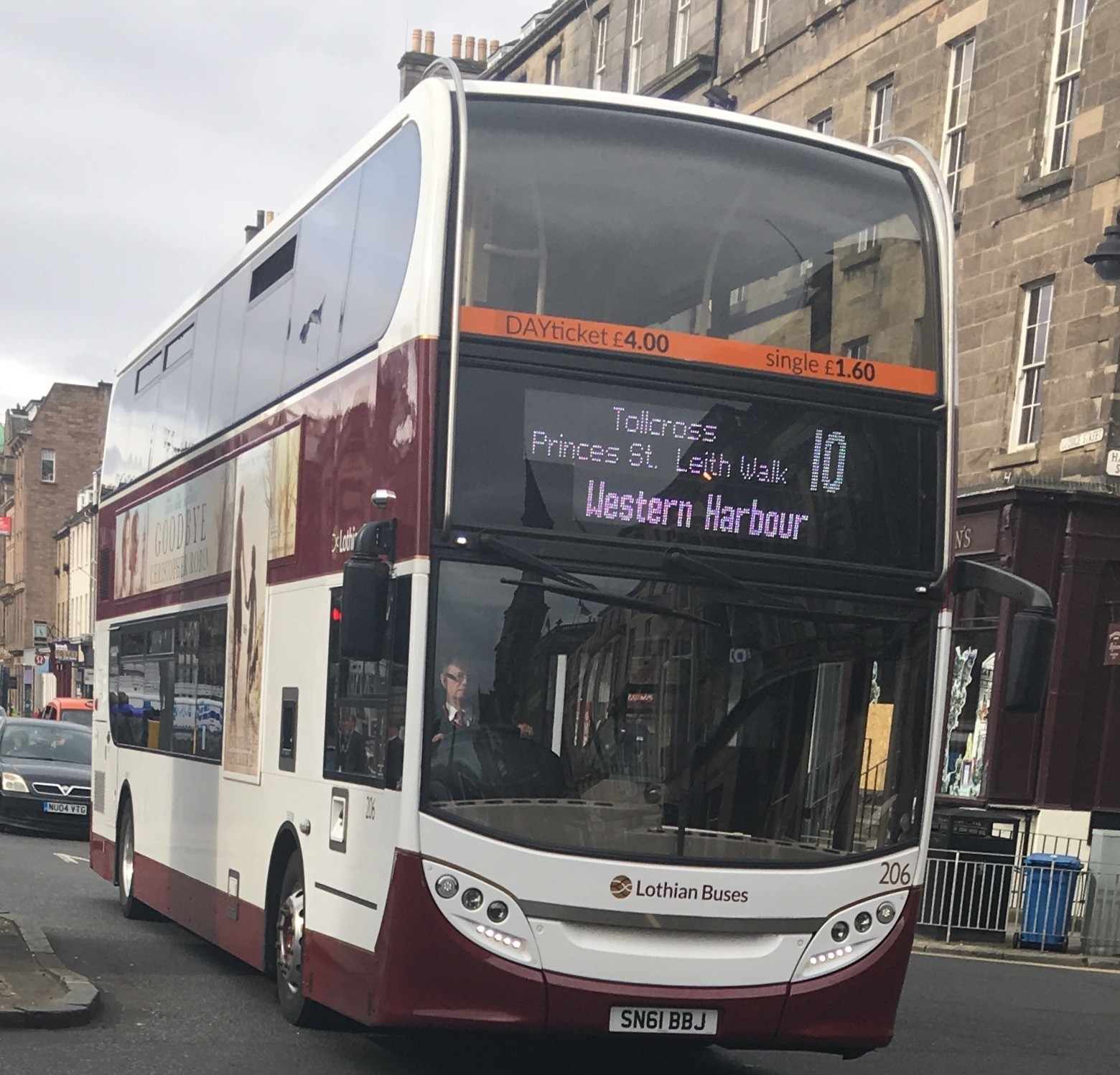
[34,698,93,729]
[1,714,92,835]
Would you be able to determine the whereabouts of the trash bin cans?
[914,809,1019,942]
[1014,852,1083,953]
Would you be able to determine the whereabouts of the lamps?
[702,84,738,109]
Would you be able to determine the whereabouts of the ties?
[457,710,464,727]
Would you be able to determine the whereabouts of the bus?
[87,73,1053,1056]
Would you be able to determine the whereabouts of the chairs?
[8,731,29,750]
[62,739,88,759]
[64,712,80,723]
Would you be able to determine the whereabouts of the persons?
[422,656,534,760]
[5,731,41,758]
[110,691,150,746]
[335,713,370,775]
[384,722,404,789]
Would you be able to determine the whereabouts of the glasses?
[446,672,482,684]
